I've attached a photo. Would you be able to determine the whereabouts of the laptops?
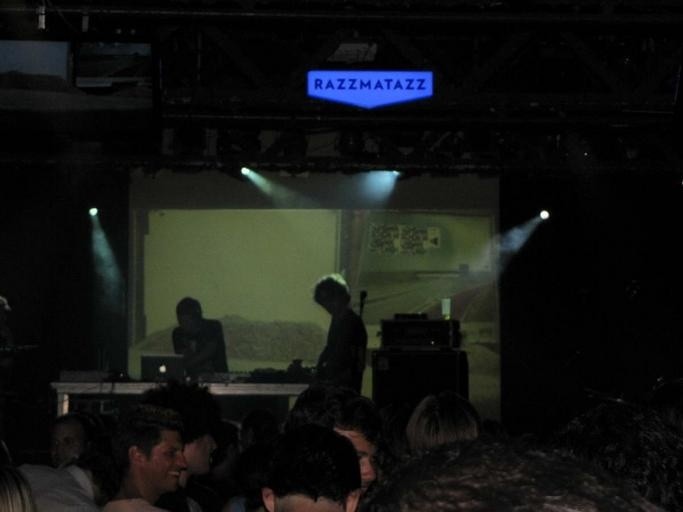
[139,354,184,384]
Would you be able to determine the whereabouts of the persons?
[307,274,368,394]
[170,295,230,379]
[0,375,683,510]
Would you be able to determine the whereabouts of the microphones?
[358,291,367,309]
